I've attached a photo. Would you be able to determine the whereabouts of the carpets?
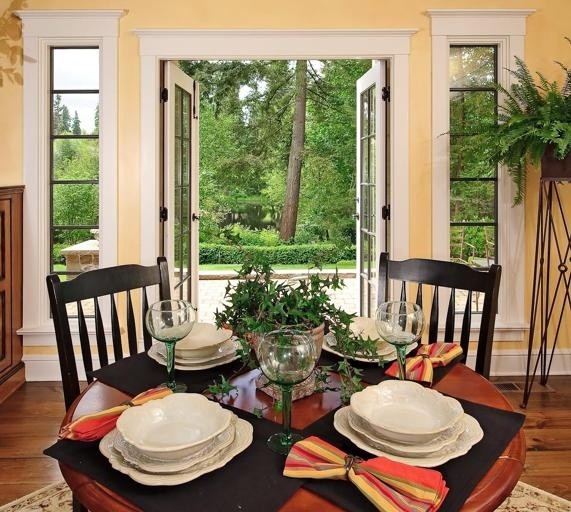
[1,479,571,511]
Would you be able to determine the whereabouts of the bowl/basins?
[350,378,466,445]
[116,392,234,461]
[329,316,404,347]
[160,322,235,359]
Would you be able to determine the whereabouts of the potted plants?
[437,37,571,208]
[209,259,391,422]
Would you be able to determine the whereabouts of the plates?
[111,423,238,474]
[346,406,469,455]
[147,335,253,371]
[326,333,398,356]
[155,337,240,364]
[333,403,487,469]
[98,413,255,488]
[322,331,420,365]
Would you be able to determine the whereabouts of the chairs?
[377,253,502,381]
[46,255,174,407]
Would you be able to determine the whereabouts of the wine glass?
[145,299,196,393]
[257,329,318,457]
[376,300,427,380]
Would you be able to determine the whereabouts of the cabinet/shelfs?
[520,176,571,410]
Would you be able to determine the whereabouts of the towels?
[283,434,452,511]
[58,387,174,442]
